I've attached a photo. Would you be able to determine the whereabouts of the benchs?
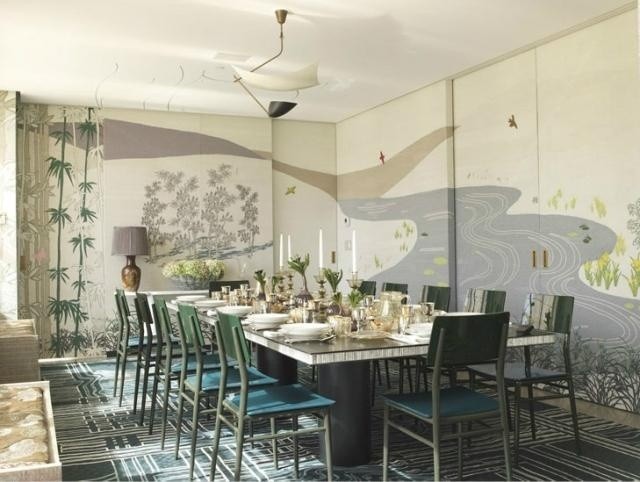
[0,381,62,482]
[0,319,40,383]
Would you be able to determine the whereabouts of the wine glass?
[351,307,365,331]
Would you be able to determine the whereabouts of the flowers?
[162,260,226,282]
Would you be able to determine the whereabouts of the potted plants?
[253,270,266,300]
[287,252,314,301]
[322,266,345,316]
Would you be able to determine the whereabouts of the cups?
[228,295,237,306]
[240,283,249,298]
[253,299,266,314]
[364,292,438,331]
[221,285,230,296]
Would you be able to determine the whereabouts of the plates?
[349,329,392,342]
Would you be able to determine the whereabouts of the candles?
[279,232,284,269]
[288,232,291,276]
[352,229,357,274]
[319,227,324,269]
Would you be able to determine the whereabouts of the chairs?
[149,295,240,451]
[465,293,575,445]
[355,281,508,432]
[210,311,337,482]
[114,289,212,426]
[378,310,511,482]
[175,302,277,480]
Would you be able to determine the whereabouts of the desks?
[160,297,557,468]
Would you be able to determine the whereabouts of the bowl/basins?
[278,322,332,339]
[246,312,289,325]
[175,295,227,309]
[216,305,253,317]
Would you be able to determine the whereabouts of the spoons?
[286,334,335,343]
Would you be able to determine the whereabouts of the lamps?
[111,227,149,293]
[234,10,297,117]
